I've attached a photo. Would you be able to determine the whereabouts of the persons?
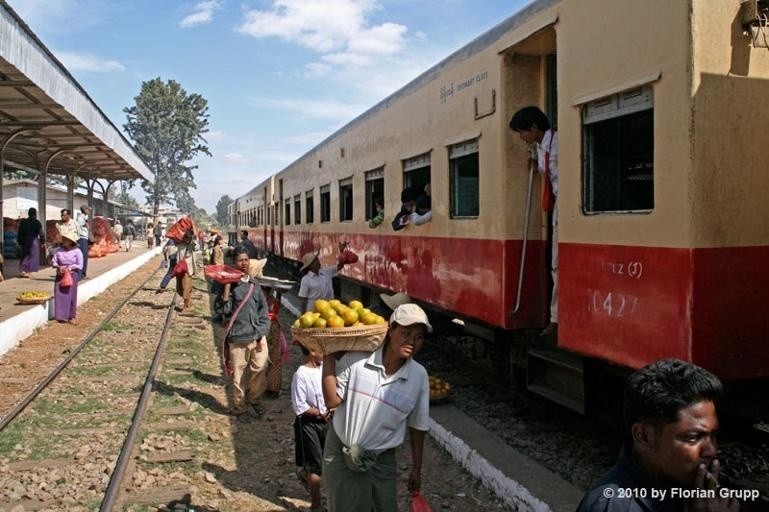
[112,219,124,248]
[576,359,740,510]
[145,211,261,314]
[321,303,434,510]
[702,466,722,491]
[123,219,137,252]
[290,338,336,510]
[16,207,46,278]
[391,182,432,231]
[56,208,81,240]
[214,247,271,422]
[510,104,559,343]
[51,230,85,324]
[75,205,93,280]
[248,256,288,399]
[367,291,416,328]
[367,195,384,230]
[298,239,353,324]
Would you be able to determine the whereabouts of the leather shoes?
[251,404,264,415]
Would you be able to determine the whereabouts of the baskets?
[430,396,450,406]
[15,291,52,306]
[203,264,246,284]
[210,231,218,235]
[289,321,390,356]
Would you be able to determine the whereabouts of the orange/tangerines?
[292,299,384,329]
[16,291,49,298]
[429,376,455,399]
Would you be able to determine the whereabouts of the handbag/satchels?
[59,269,73,289]
[221,326,232,378]
[338,243,358,264]
[543,175,556,211]
[174,261,187,278]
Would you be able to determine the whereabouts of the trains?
[227,0,768,420]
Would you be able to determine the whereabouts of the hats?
[81,205,90,210]
[389,302,434,333]
[300,248,321,272]
[248,257,268,278]
[59,229,81,247]
[379,292,413,311]
